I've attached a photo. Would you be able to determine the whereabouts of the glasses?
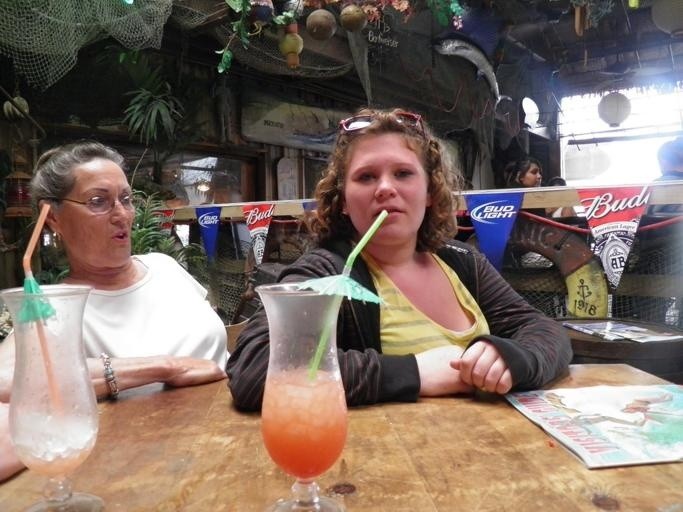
[41,194,137,213]
[338,113,425,144]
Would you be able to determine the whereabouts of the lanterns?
[598,92,631,127]
[652,0,683,39]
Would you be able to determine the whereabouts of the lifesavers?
[465,222,614,320]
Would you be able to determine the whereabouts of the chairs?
[225,318,250,357]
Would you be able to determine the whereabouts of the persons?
[223,107,573,414]
[504,156,542,187]
[544,176,576,219]
[650,141,683,211]
[0,403,26,482]
[0,138,232,403]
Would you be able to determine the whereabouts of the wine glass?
[0,285,105,511]
[254,275,348,511]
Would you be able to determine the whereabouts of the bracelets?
[101,352,119,399]
